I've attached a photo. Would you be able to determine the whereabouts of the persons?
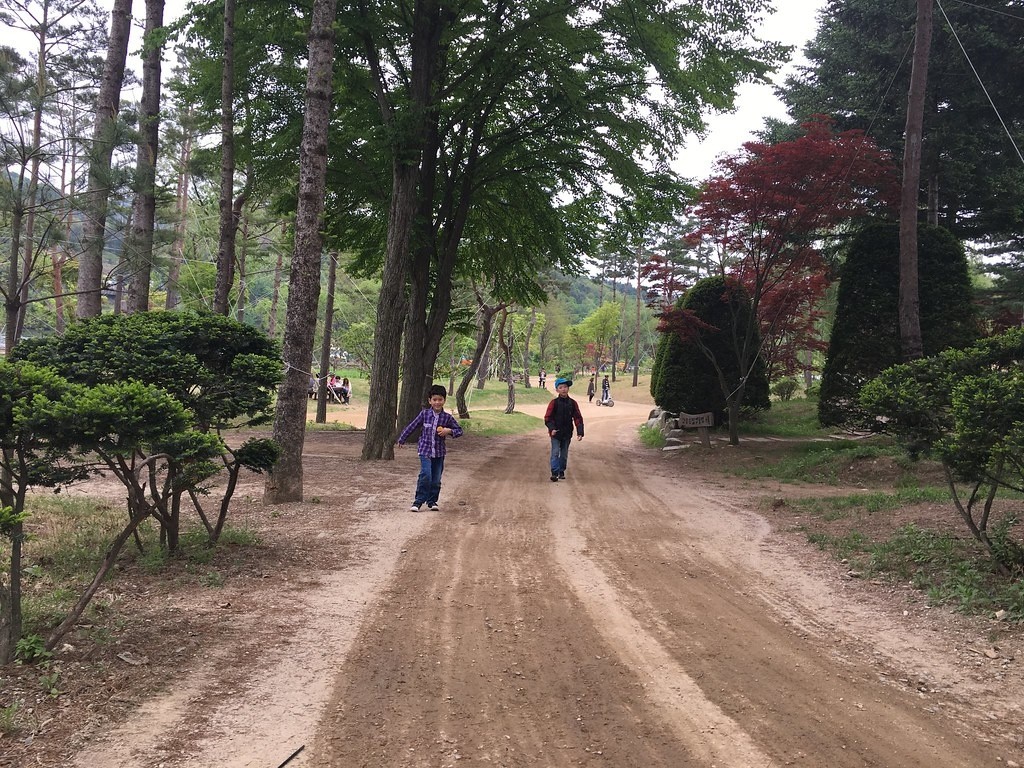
[545,379,586,481]
[306,371,352,404]
[538,368,611,401]
[399,385,465,512]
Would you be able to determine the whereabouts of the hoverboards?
[596,388,615,407]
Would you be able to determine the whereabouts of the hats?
[555,378,573,389]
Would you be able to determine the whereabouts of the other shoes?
[557,475,565,479]
[428,502,439,510]
[549,476,558,482]
[410,500,422,511]
